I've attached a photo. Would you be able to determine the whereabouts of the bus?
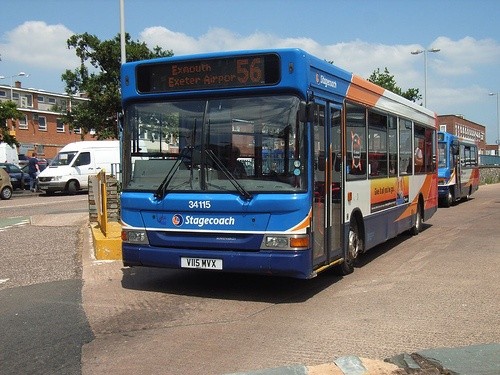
[114,47,439,281]
[437,131,480,207]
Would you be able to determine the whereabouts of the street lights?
[0,72,26,102]
[411,48,440,108]
[0,72,26,80]
[488,92,500,145]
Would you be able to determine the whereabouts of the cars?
[0,157,53,200]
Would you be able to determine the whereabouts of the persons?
[225,147,247,179]
[28,152,41,195]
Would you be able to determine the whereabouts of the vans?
[34,138,151,196]
